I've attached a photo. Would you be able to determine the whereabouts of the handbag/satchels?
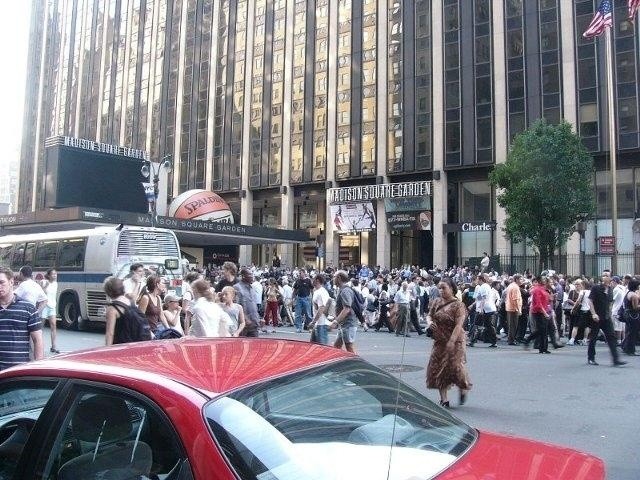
[563,300,575,310]
[618,305,626,322]
[572,303,582,316]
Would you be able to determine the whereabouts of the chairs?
[57,394,153,480]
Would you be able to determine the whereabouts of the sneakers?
[363,325,434,337]
[464,331,588,353]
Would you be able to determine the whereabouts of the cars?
[0,335,606,479]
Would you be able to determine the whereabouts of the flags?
[627,0,640,24]
[582,0,613,41]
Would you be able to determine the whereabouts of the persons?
[39,270,61,354]
[0,267,44,372]
[103,261,640,407]
[12,266,48,360]
[334,204,377,230]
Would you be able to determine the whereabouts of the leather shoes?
[588,360,599,366]
[614,361,630,367]
[459,391,468,405]
[437,401,451,409]
[627,350,639,357]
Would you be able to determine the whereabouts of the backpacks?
[107,297,151,344]
[340,286,366,314]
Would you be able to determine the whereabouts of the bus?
[2,219,183,330]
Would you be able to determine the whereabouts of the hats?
[164,293,182,304]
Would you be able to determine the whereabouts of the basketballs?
[169,189,234,224]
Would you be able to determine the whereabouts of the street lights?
[140,152,174,227]
